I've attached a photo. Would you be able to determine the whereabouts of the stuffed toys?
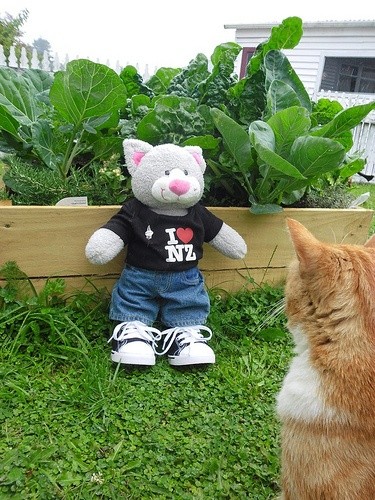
[83,138,247,367]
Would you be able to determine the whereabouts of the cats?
[274,216,375,499]
